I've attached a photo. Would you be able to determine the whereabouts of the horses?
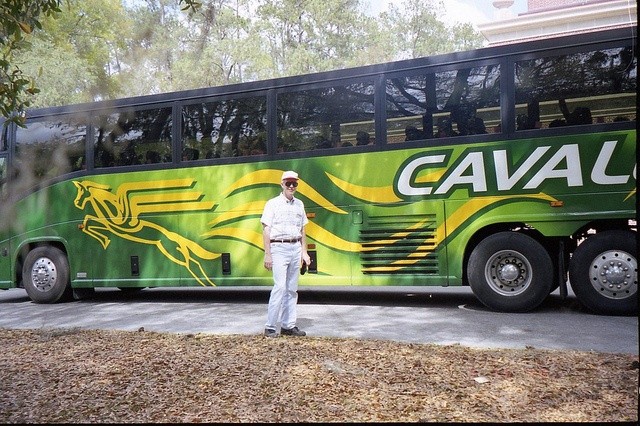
[71,180,223,288]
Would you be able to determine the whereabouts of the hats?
[282,171,299,181]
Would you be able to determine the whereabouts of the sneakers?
[281,327,306,336]
[265,329,277,338]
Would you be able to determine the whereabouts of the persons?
[261,170,311,338]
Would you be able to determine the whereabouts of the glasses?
[283,182,298,187]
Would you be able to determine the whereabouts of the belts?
[271,238,300,243]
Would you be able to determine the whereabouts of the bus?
[0,26,640,316]
